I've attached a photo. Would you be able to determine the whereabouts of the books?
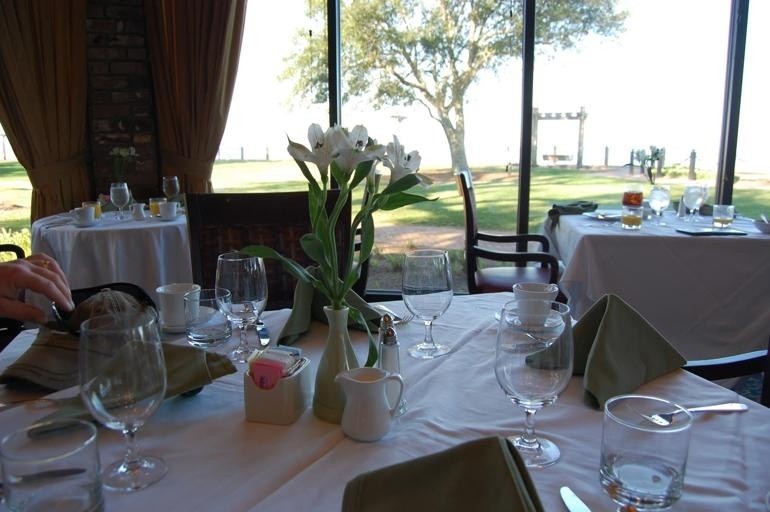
[674,227,748,236]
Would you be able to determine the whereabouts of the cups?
[335,367,402,442]
[73,207,96,224]
[512,281,560,327]
[1,418,105,512]
[713,204,735,228]
[600,393,693,511]
[149,197,168,217]
[82,202,103,222]
[159,201,180,219]
[130,203,147,219]
[156,280,199,327]
[184,288,232,347]
[622,185,643,205]
[622,205,643,230]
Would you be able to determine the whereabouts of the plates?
[501,298,571,331]
[582,210,625,221]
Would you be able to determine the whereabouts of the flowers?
[232,123,440,367]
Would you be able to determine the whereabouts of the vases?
[312,305,360,424]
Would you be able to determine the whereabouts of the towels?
[674,201,736,217]
[41,213,73,228]
[549,201,599,232]
[523,291,689,411]
[166,192,187,204]
[278,268,404,346]
[342,436,546,512]
[101,190,149,212]
[31,340,237,439]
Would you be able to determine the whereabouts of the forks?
[639,402,748,427]
[374,303,415,327]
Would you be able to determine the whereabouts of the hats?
[0,288,159,391]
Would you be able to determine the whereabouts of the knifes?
[0,466,86,486]
[252,303,270,346]
[558,485,593,512]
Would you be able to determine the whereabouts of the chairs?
[681,332,770,412]
[0,244,26,331]
[183,190,352,311]
[352,173,382,298]
[454,168,568,304]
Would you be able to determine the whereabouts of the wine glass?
[649,187,671,225]
[495,300,572,466]
[402,248,456,360]
[163,175,181,204]
[109,182,129,220]
[215,251,268,361]
[693,185,707,222]
[78,312,164,490]
[683,183,703,223]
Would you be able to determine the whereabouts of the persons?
[0,254,75,324]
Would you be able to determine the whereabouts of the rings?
[42,260,50,267]
